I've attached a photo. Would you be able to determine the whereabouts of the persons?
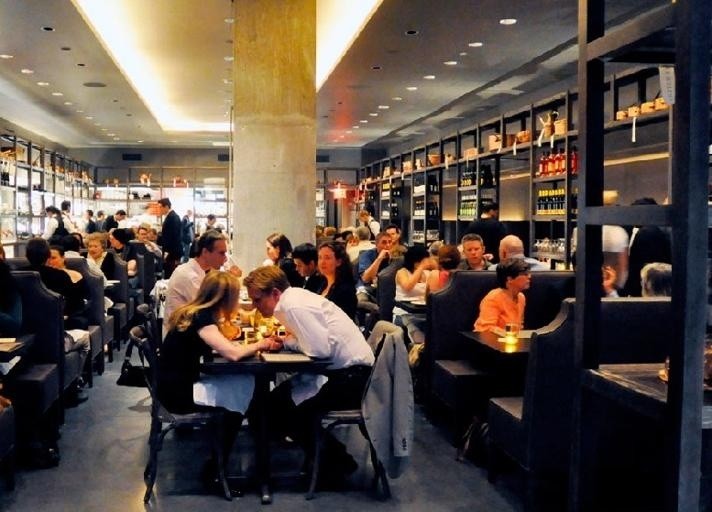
[267,232,294,276]
[162,272,284,496]
[290,241,318,293]
[244,265,376,477]
[315,201,672,342]
[161,230,229,327]
[315,243,357,316]
[1,198,223,383]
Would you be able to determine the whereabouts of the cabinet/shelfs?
[0,127,227,276]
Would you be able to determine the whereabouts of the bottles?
[52,156,94,185]
[1,160,11,187]
[535,146,577,176]
[378,181,401,217]
[414,199,439,215]
[459,193,494,219]
[460,163,494,187]
[412,173,438,194]
[535,188,581,216]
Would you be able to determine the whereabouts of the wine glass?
[260,318,274,338]
[534,237,575,254]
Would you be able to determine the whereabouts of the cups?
[505,320,521,344]
[616,96,670,120]
[604,189,618,207]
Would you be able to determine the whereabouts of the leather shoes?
[325,456,358,479]
[289,467,324,484]
[198,479,242,496]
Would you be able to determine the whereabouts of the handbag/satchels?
[117,361,154,387]
